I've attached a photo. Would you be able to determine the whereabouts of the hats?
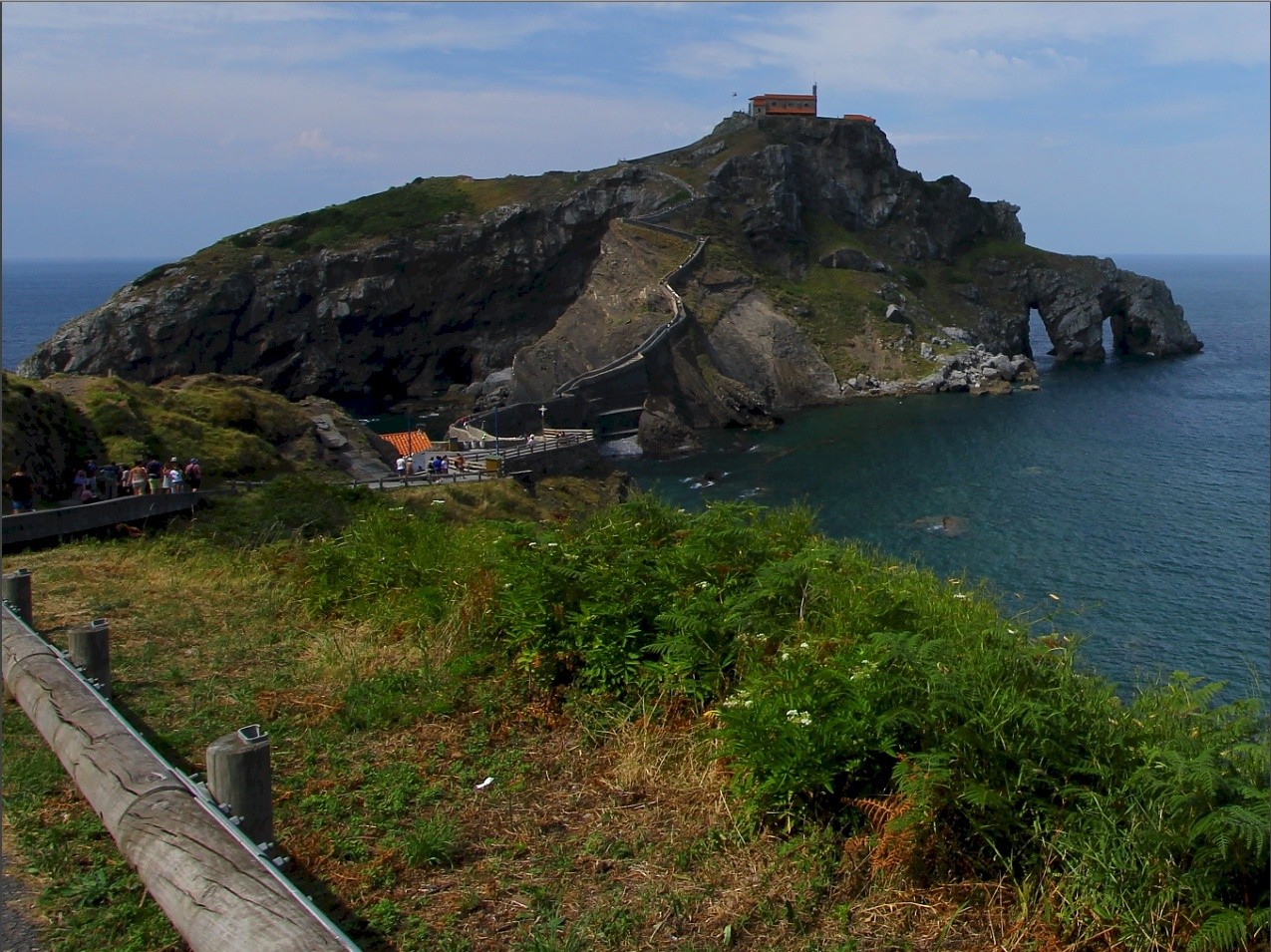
[170,457,177,462]
[190,458,198,463]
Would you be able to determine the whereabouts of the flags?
[732,91,737,96]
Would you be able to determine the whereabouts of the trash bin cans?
[448,437,459,452]
[205,723,272,850]
[463,439,469,451]
[485,455,502,477]
[2,568,33,629]
[479,439,485,449]
[65,617,113,702]
[471,439,477,449]
[507,470,536,498]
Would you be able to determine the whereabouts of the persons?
[396,452,466,482]
[464,419,468,430]
[6,464,35,514]
[75,456,202,504]
[527,434,534,448]
[554,430,567,447]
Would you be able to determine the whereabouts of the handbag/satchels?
[186,468,197,488]
